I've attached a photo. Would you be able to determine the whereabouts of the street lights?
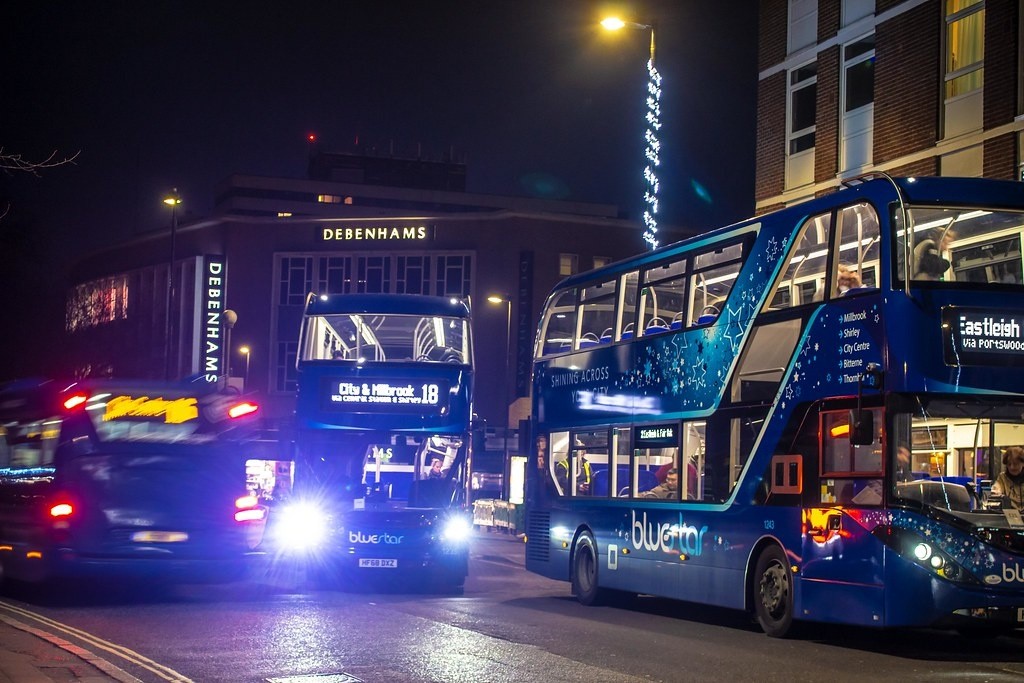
[487,293,512,502]
[239,346,250,394]
[600,14,660,254]
[218,308,238,395]
[163,187,182,384]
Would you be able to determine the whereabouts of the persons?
[651,469,692,500]
[975,450,990,476]
[333,349,344,360]
[655,452,696,490]
[910,227,950,280]
[990,447,1024,514]
[812,265,868,300]
[557,440,592,495]
[868,442,916,502]
[425,458,443,478]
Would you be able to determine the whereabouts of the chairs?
[670,311,697,331]
[622,323,635,339]
[580,332,600,349]
[599,328,612,343]
[698,305,720,325]
[560,342,573,352]
[644,317,670,334]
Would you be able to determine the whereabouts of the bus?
[281,291,472,598]
[519,169,1022,655]
[1,375,271,596]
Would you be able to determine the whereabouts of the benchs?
[910,471,930,480]
[924,475,982,512]
[852,479,867,497]
[592,468,658,498]
[554,461,663,473]
